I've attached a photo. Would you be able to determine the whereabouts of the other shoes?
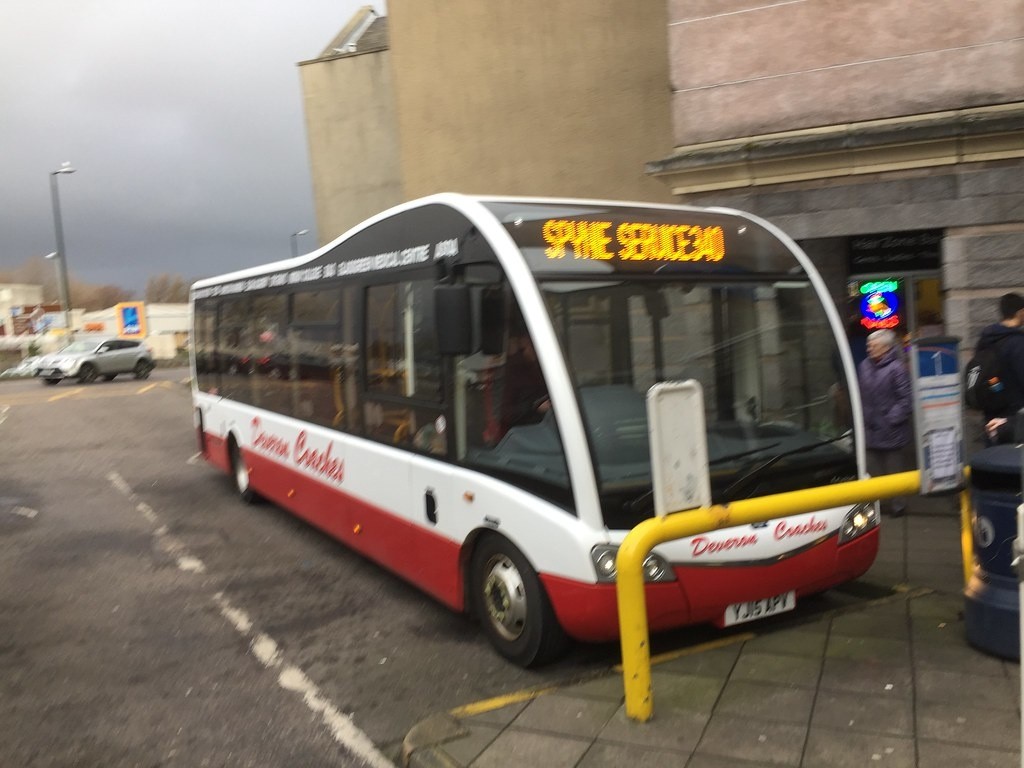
[891,502,904,516]
[880,501,891,513]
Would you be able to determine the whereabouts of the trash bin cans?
[961,443,1024,662]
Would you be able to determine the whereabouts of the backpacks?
[964,334,1015,408]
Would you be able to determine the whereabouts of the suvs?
[29,337,158,384]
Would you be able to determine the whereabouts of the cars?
[194,349,252,374]
[293,371,484,438]
[243,345,345,383]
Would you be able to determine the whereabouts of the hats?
[1001,294,1024,314]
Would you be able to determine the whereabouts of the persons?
[966,290,1024,449]
[197,367,448,454]
[856,330,914,515]
[492,330,550,431]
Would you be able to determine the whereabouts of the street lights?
[50,167,77,343]
[290,228,308,256]
[46,250,69,347]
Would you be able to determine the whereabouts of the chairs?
[234,357,410,445]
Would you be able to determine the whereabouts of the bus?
[187,191,881,667]
